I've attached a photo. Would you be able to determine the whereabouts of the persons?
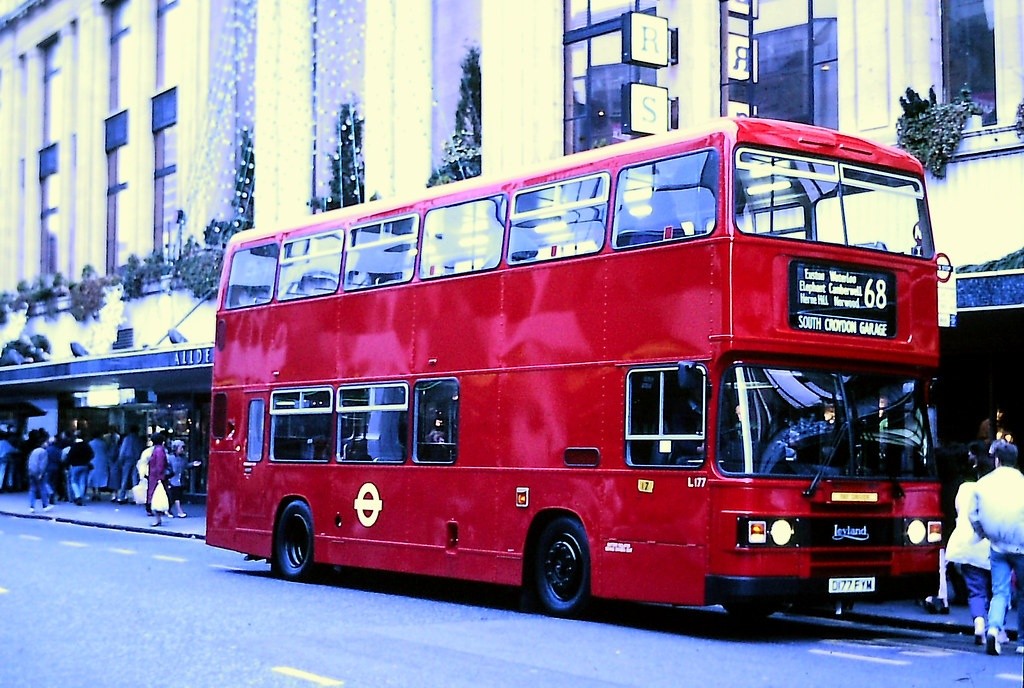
[933,439,1024,657]
[0,423,201,528]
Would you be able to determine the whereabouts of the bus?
[204,116,943,618]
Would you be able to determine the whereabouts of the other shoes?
[146,510,154,516]
[32,488,136,507]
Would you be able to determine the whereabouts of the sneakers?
[997,627,1009,645]
[177,512,187,518]
[29,507,34,513]
[972,617,987,645]
[42,504,53,512]
[1015,645,1023,654]
[982,630,1000,655]
[164,510,174,518]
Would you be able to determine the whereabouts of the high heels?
[149,519,162,528]
